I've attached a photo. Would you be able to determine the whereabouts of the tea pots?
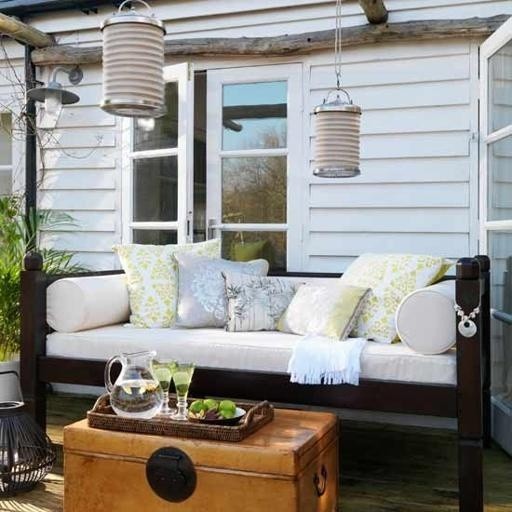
[104,350,165,421]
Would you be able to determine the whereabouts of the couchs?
[21,253,490,511]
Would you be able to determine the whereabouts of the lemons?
[190,399,236,418]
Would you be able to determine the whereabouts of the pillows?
[280,283,374,342]
[218,269,307,334]
[173,253,270,330]
[112,238,223,328]
[341,254,453,345]
[46,273,130,332]
[394,279,457,353]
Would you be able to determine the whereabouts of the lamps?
[99,0,166,120]
[26,67,80,133]
[310,1,363,178]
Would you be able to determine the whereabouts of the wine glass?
[170,362,195,422]
[153,367,177,416]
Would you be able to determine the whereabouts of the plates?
[186,405,247,427]
